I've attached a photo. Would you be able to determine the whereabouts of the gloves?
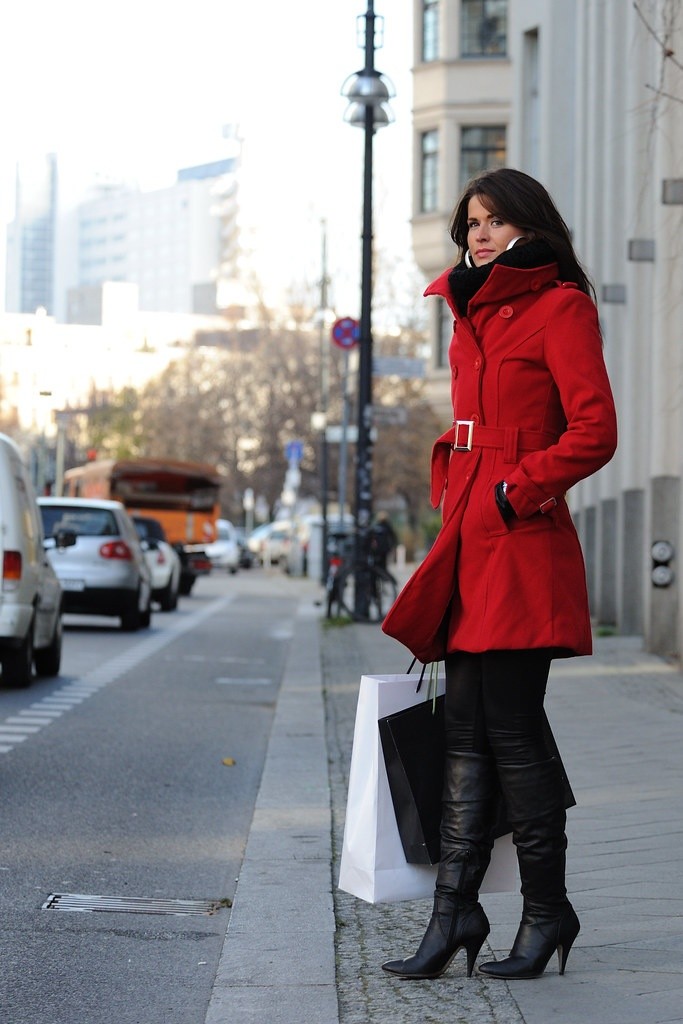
[494,480,514,522]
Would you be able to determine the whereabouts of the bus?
[44,456,224,598]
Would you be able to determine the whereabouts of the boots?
[381,749,503,979]
[478,757,580,979]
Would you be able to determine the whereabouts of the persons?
[381,169,618,978]
[375,512,396,565]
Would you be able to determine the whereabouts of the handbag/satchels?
[378,693,576,866]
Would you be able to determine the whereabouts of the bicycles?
[324,529,398,624]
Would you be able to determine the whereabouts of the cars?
[35,495,161,633]
[129,513,184,612]
[210,512,353,575]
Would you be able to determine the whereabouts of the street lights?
[344,0,400,626]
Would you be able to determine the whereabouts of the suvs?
[1,434,79,689]
